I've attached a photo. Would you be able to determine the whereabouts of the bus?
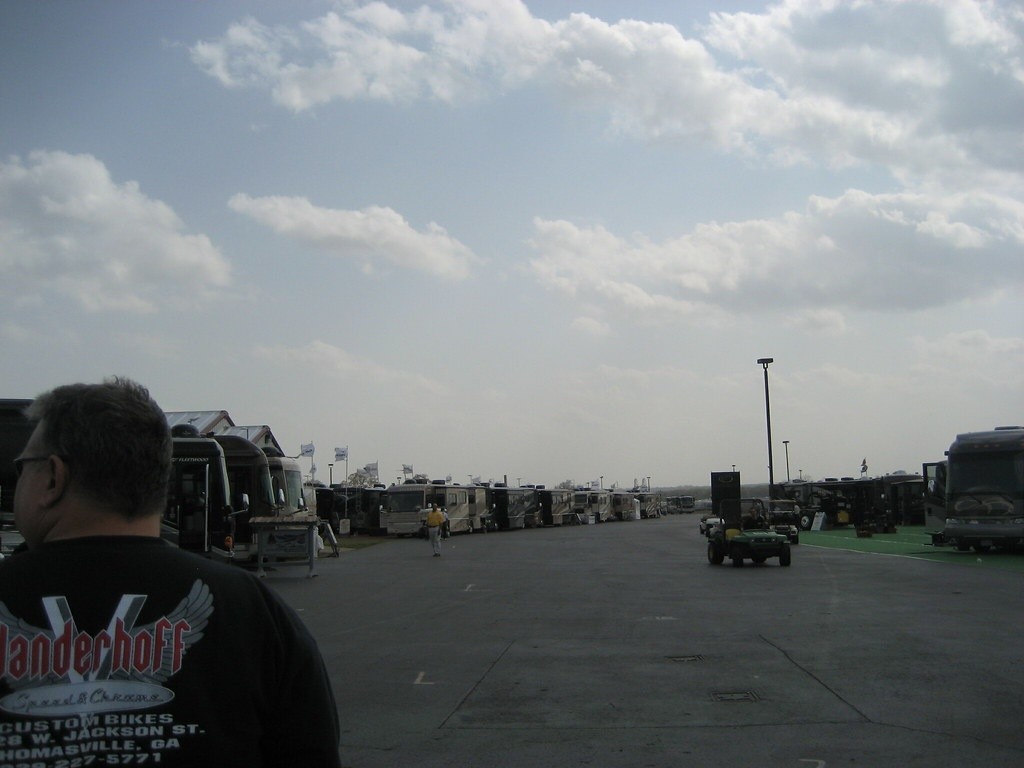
[769,426,1024,553]
[0,397,661,571]
[666,495,695,514]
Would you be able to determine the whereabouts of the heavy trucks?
[710,471,741,516]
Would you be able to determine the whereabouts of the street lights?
[732,464,735,472]
[799,470,802,479]
[782,440,790,481]
[328,463,333,488]
[757,357,774,496]
[600,475,603,490]
[467,474,472,485]
[647,477,651,492]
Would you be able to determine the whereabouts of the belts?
[428,525,439,528]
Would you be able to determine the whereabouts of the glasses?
[13,455,73,477]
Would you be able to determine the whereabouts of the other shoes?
[433,553,440,557]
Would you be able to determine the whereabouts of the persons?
[0,376,341,767]
[743,508,763,529]
[440,507,447,539]
[426,503,442,557]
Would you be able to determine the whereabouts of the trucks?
[699,499,800,568]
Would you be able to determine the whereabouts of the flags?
[366,463,377,474]
[309,463,315,474]
[300,444,314,457]
[334,447,348,461]
[403,465,412,474]
